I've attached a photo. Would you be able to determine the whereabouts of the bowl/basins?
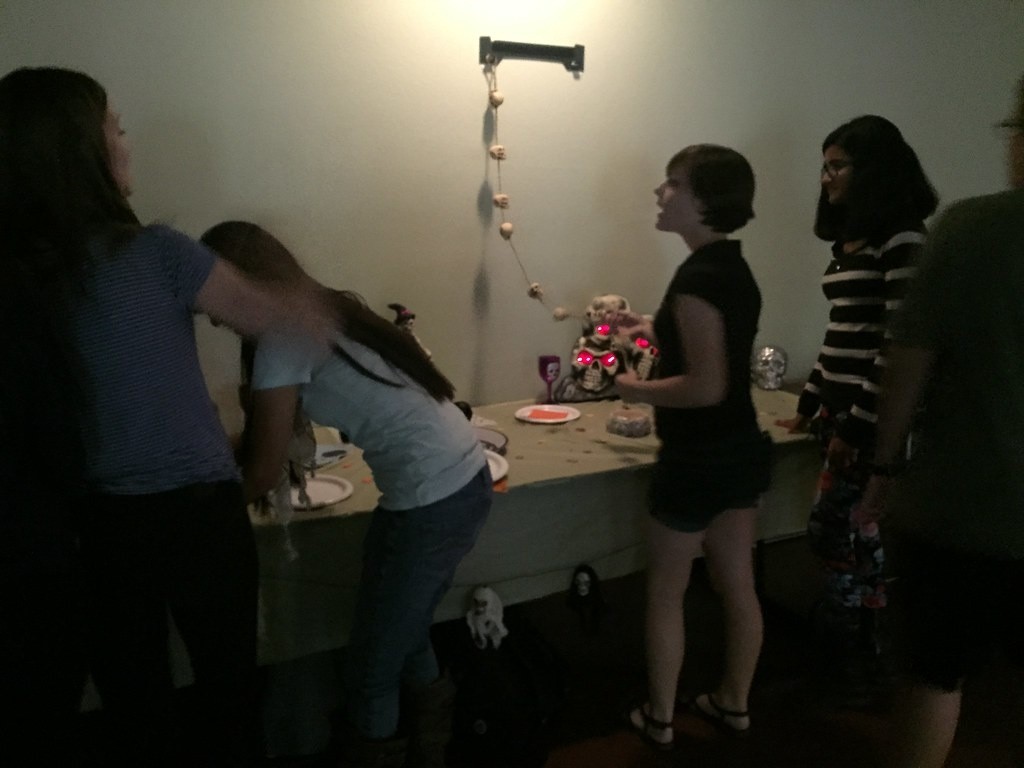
[473,425,508,454]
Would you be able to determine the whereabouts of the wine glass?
[539,355,562,404]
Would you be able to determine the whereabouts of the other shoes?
[831,559,906,607]
[809,597,881,631]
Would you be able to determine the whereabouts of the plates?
[290,474,354,507]
[515,405,581,423]
[482,448,508,482]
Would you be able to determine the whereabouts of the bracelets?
[871,462,904,478]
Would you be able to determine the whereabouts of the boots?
[334,714,409,768]
[402,669,457,768]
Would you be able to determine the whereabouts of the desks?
[81,388,821,709]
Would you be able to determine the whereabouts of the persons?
[0,66,278,768]
[775,116,936,663]
[615,145,770,748]
[852,70,1024,768]
[197,222,494,767]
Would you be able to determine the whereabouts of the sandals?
[676,690,754,737]
[611,692,675,751]
